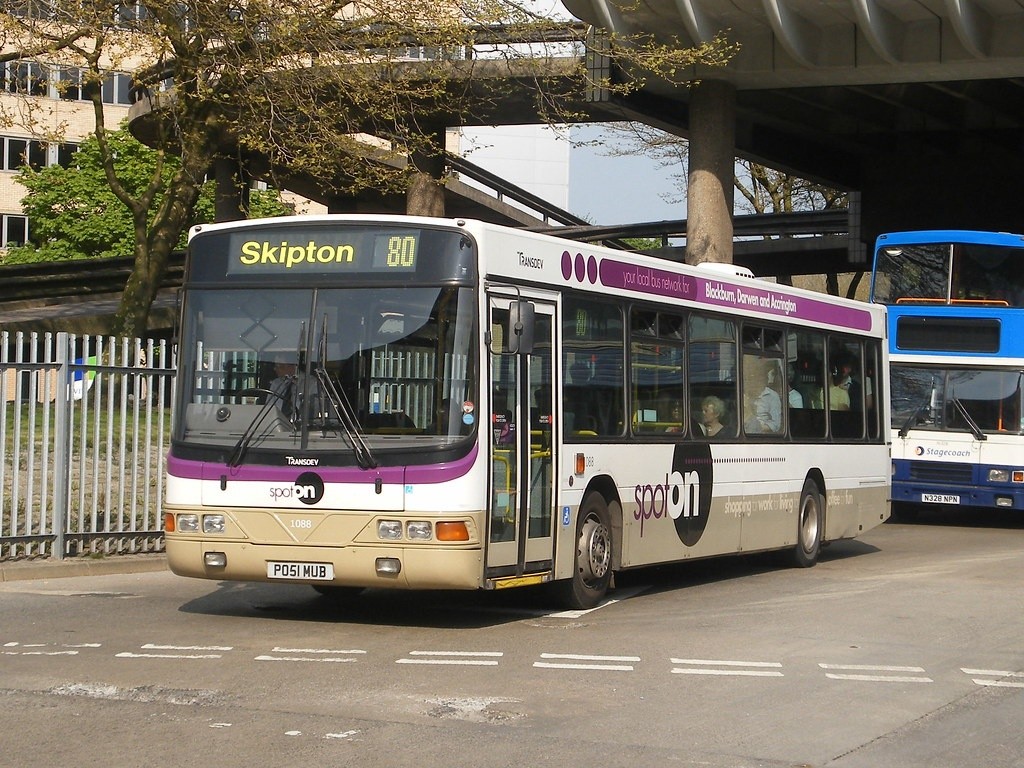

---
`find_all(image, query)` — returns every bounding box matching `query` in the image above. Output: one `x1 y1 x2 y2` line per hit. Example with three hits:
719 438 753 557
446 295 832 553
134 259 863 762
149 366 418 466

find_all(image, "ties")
281 382 293 419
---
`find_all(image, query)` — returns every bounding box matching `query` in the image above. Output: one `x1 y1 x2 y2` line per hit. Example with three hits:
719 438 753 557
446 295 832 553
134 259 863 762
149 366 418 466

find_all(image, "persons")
425 398 468 435
266 352 320 419
701 396 735 437
564 359 616 435
789 359 872 410
664 398 704 435
744 368 781 433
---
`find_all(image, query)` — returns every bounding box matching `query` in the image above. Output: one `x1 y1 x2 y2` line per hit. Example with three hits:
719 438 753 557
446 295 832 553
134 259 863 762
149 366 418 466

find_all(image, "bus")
867 228 1024 513
159 212 894 613
